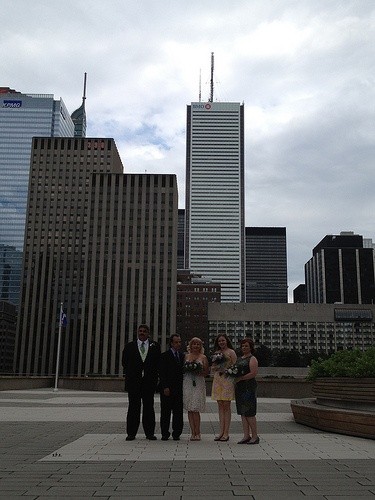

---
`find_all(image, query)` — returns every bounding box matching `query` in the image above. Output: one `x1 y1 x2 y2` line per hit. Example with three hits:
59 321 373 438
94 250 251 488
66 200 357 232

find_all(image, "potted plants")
306 347 375 406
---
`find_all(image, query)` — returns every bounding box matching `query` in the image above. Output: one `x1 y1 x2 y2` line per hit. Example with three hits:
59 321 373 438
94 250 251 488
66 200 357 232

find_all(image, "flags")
62 309 68 326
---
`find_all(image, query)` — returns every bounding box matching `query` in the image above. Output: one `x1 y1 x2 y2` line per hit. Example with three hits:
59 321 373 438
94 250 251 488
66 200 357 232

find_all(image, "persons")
183 337 209 440
235 338 260 445
160 334 186 441
122 325 160 441
211 334 237 442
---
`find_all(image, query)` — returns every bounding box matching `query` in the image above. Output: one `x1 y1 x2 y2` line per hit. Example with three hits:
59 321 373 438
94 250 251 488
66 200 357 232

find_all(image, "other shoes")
173 436 180 440
219 435 229 441
246 437 259 444
190 435 196 441
126 434 135 440
195 435 201 441
161 436 169 440
237 436 251 444
214 434 223 441
146 434 157 440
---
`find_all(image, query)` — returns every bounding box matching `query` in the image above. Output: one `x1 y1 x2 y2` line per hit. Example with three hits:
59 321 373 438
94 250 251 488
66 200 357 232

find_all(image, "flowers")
184 359 202 373
211 351 229 369
150 342 154 347
221 362 247 382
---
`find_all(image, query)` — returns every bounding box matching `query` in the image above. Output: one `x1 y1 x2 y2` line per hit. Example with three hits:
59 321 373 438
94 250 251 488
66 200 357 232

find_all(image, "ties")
174 352 179 361
140 343 145 354
